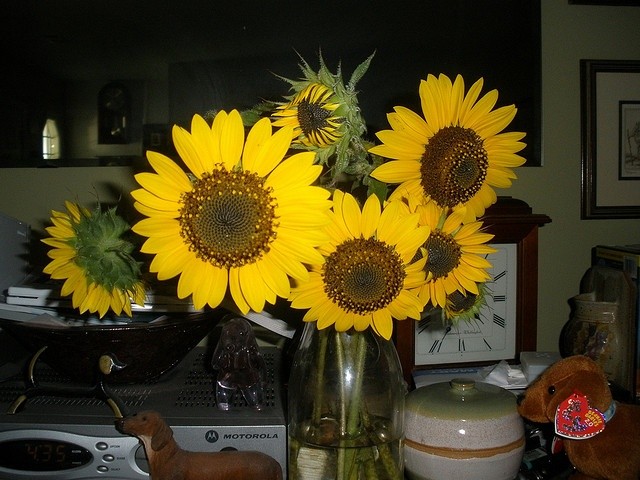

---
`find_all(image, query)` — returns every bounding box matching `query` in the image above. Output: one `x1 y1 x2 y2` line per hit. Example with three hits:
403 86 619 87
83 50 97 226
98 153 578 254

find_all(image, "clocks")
401 191 550 377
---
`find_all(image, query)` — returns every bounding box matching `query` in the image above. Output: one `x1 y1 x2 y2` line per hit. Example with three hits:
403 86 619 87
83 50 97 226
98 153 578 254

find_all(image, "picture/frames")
579 60 638 221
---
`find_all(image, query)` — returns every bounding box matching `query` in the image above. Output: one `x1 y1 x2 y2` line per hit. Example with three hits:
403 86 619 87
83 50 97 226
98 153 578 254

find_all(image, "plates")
0 308 225 383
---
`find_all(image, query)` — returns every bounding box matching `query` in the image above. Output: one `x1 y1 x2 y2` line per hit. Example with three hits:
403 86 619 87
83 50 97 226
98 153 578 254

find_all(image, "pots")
404 378 525 480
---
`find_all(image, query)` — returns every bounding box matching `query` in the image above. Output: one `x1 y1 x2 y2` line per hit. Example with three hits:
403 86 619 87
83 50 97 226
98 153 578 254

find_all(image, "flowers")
45 42 528 466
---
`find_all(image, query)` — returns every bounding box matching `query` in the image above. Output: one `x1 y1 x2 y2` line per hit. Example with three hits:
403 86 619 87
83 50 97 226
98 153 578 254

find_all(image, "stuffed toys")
517 354 639 479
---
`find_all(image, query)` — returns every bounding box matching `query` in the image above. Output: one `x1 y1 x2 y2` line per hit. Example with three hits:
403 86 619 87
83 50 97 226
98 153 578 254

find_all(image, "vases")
285 308 406 480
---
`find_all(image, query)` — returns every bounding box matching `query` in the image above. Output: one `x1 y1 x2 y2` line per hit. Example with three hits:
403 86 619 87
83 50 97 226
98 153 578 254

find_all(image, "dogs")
212 319 266 410
114 410 283 480
